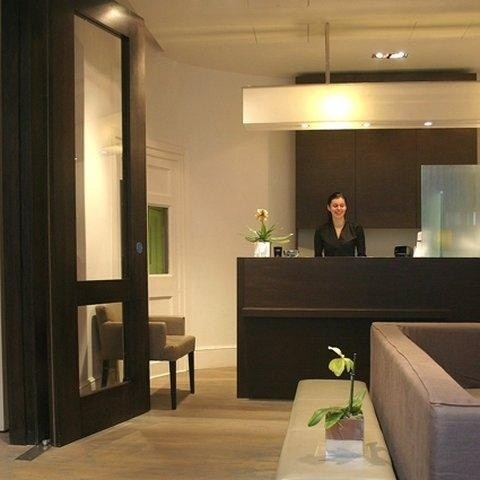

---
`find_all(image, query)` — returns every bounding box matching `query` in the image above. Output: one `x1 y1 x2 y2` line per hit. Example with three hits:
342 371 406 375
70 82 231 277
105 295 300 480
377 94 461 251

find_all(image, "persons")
314 192 366 257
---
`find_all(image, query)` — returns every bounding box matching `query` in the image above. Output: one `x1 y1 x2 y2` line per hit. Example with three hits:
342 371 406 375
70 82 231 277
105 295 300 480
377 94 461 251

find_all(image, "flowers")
237 208 294 244
308 345 367 429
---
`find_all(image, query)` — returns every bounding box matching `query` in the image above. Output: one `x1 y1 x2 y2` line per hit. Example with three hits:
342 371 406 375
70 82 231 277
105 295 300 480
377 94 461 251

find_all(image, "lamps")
102 143 124 156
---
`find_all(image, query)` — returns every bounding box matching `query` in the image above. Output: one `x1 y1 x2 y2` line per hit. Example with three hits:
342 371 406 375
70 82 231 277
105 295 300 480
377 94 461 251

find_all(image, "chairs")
95 302 196 408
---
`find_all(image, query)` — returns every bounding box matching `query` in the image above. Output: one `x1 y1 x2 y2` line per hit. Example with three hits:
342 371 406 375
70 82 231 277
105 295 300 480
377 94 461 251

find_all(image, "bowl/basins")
283 249 301 258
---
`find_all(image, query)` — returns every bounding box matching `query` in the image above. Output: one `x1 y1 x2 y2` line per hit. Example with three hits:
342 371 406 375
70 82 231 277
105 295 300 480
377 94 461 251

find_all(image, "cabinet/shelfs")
294 70 478 227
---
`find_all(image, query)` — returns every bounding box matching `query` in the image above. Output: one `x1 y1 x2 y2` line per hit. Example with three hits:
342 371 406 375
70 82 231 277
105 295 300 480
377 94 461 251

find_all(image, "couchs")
369 321 480 480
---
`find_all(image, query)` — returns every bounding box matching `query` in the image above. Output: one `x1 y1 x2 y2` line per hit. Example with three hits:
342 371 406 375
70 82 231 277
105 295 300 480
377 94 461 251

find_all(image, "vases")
253 242 271 258
324 415 364 464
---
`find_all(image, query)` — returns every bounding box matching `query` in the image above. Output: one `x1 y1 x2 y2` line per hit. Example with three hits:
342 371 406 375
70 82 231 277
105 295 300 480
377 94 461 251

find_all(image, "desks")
276 380 397 480
